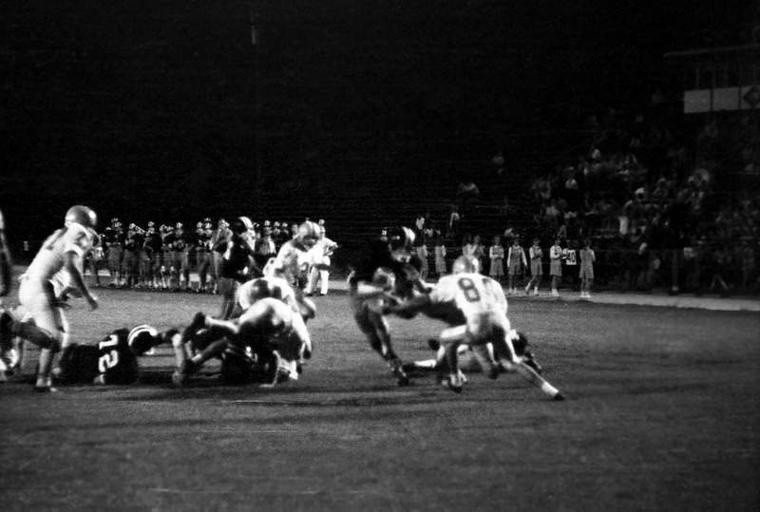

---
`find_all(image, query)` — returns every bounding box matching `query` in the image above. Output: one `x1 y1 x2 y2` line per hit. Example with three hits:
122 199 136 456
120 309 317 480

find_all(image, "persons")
345 225 565 402
0 203 338 394
409 148 760 299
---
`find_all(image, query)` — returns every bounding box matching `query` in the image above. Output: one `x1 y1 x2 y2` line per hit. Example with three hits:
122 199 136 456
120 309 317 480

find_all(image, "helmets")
238 216 252 229
372 266 396 289
127 324 156 355
452 254 479 273
221 354 251 382
251 279 268 297
296 221 320 249
65 205 98 227
387 226 415 263
505 329 526 354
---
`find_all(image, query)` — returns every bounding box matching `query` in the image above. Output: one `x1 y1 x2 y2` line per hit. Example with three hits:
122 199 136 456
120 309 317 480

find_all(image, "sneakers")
183 312 205 343
179 360 199 374
552 392 563 400
1 309 16 349
33 386 57 392
157 329 177 344
387 339 462 392
